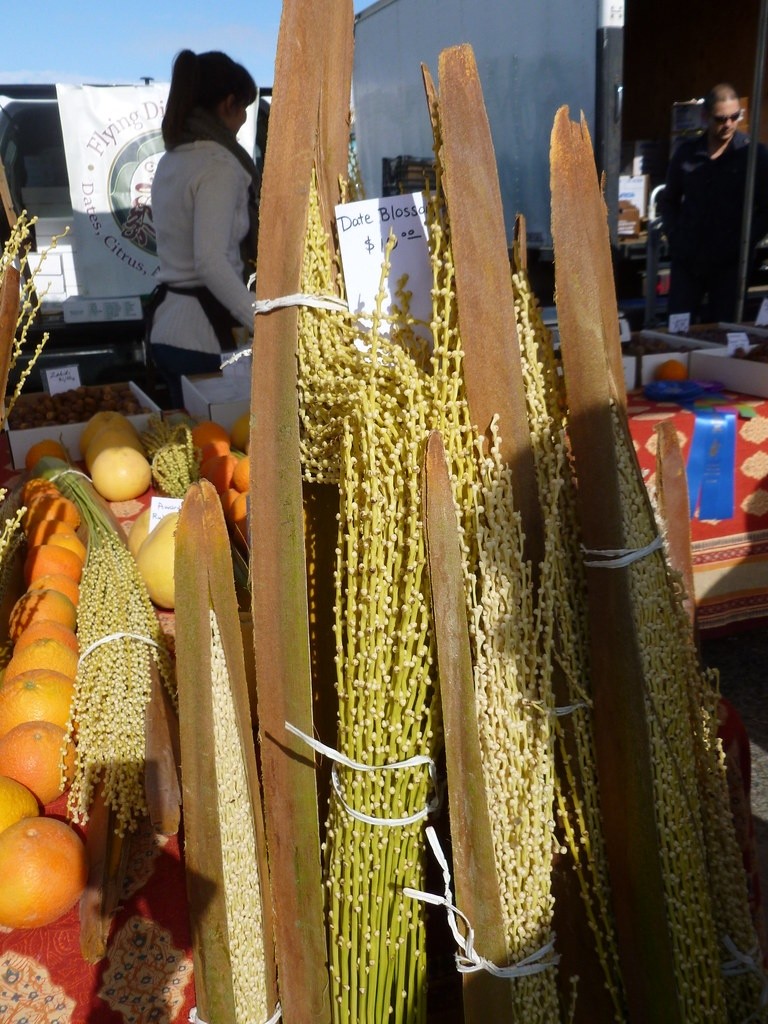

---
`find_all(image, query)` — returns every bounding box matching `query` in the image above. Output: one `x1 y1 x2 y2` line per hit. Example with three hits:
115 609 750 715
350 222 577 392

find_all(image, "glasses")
709 111 740 125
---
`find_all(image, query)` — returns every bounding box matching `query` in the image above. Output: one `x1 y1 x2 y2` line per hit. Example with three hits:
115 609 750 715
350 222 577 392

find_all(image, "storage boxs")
641 321 768 348
41 365 83 396
555 354 635 392
688 344 768 398
221 348 254 375
2 381 164 470
180 361 254 435
623 331 727 388
61 294 144 323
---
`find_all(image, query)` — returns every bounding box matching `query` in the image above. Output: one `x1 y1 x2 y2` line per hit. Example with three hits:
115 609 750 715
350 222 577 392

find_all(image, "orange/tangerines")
0 437 89 927
653 359 686 380
187 420 249 545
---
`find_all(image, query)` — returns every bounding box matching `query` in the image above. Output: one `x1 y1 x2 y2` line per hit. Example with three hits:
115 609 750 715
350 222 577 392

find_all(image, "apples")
77 412 179 609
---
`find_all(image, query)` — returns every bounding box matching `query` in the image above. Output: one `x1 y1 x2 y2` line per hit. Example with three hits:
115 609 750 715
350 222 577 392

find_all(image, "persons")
140 47 258 419
656 81 768 331
